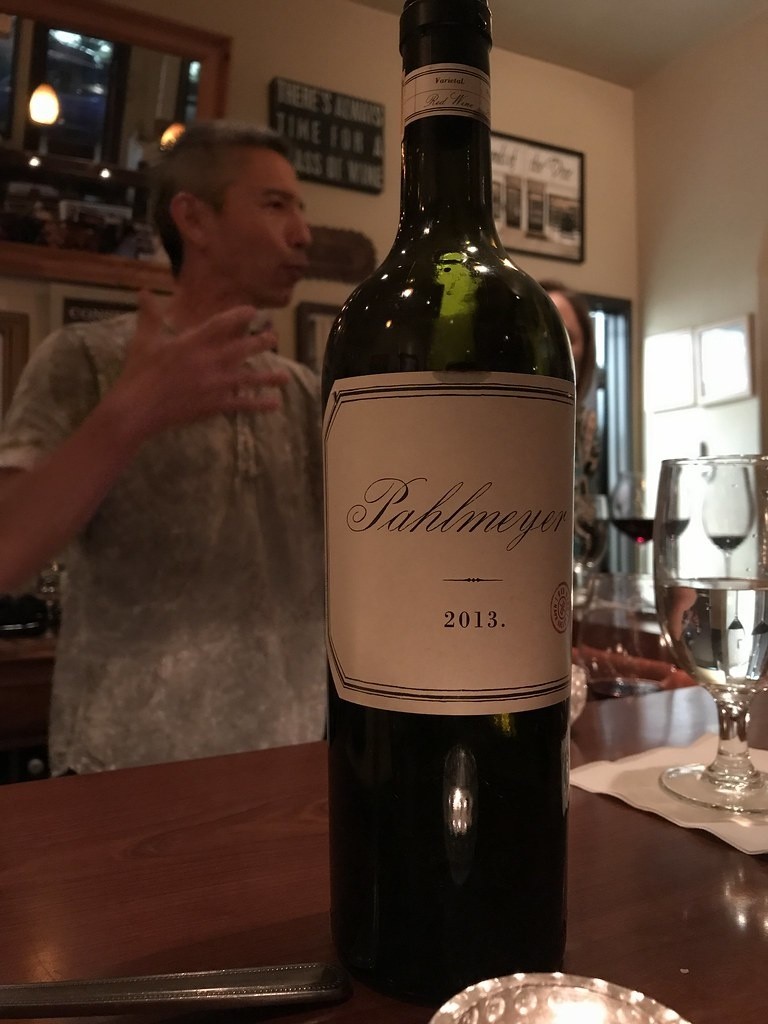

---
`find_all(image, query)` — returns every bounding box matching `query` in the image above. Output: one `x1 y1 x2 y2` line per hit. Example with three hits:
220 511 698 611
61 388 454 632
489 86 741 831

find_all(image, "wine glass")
701 468 753 580
661 483 692 584
654 453 768 813
572 492 607 588
723 590 745 664
750 590 768 657
607 471 654 574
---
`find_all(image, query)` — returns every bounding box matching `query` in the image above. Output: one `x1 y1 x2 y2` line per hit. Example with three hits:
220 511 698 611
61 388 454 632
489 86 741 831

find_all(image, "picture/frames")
647 313 753 415
492 129 585 263
1 0 234 296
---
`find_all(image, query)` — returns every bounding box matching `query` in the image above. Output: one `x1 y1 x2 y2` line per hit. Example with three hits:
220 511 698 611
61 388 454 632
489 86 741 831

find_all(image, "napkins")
568 729 767 857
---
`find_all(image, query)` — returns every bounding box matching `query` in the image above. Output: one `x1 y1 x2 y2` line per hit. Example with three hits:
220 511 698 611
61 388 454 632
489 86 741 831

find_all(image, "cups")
428 972 688 1024
578 570 669 696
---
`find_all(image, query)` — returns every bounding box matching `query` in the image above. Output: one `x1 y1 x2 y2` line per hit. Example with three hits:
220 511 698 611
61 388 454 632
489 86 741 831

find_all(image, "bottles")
329 0 577 1024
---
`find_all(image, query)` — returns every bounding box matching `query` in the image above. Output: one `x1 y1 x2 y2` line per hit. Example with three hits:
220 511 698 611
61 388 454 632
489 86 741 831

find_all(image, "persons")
530 275 603 562
0 117 334 777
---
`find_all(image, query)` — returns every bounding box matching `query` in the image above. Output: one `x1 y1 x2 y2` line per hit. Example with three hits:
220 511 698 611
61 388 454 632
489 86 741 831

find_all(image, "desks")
1 683 768 1024
